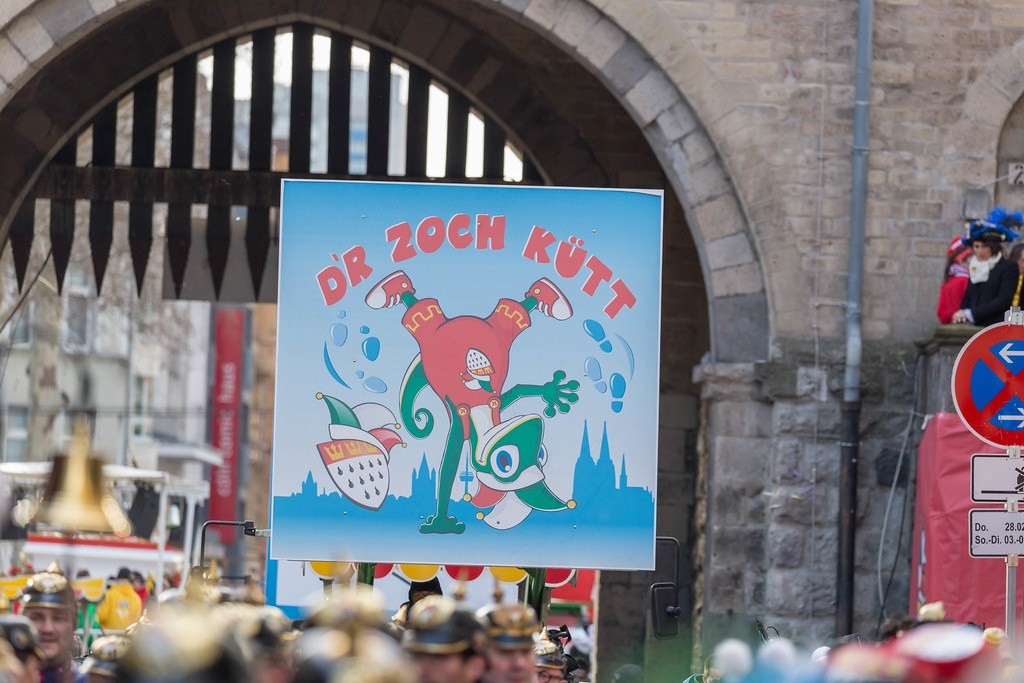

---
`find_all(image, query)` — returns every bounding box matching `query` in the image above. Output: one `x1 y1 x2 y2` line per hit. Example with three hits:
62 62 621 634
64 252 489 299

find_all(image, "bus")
0 460 197 606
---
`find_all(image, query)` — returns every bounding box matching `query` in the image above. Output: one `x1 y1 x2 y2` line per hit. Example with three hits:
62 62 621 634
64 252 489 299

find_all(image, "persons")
0 566 594 683
935 207 1024 324
686 598 1024 683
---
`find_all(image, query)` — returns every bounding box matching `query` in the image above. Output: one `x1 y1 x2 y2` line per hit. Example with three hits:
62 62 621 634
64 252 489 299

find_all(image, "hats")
124 558 281 683
17 561 76 608
401 594 483 653
487 603 541 650
530 626 565 668
0 615 38 662
291 584 422 683
408 576 444 601
960 207 1022 242
947 237 973 263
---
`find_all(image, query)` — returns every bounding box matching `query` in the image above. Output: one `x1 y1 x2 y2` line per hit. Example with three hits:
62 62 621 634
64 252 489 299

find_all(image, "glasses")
534 670 569 683
